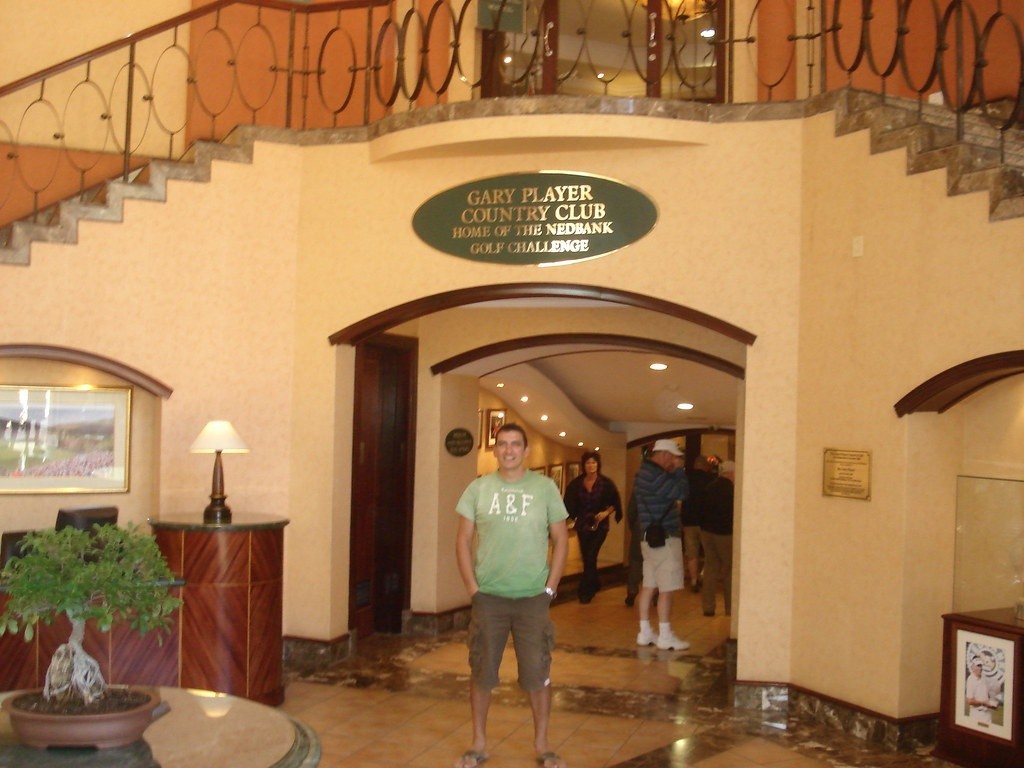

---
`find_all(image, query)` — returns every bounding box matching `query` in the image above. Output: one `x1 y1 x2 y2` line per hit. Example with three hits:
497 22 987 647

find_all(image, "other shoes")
625 593 637 606
651 592 660 606
692 580 700 592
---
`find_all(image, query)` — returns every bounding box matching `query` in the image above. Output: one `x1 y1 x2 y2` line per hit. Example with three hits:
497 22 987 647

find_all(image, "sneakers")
656 635 691 651
637 629 659 645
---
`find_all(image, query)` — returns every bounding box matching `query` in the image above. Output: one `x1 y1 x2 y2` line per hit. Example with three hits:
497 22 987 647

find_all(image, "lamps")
189 419 250 523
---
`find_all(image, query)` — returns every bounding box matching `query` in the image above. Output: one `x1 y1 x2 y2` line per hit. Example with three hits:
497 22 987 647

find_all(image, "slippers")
457 749 489 768
537 751 562 768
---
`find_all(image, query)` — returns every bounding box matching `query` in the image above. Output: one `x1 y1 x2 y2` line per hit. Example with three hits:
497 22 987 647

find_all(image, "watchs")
545 585 557 600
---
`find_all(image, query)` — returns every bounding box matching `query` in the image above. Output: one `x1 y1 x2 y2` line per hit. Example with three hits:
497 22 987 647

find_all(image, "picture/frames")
529 466 545 477
566 462 583 488
486 409 507 448
0 385 134 495
547 463 563 495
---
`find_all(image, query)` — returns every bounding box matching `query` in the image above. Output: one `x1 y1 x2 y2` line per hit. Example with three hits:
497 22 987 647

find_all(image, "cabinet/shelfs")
929 605 1024 768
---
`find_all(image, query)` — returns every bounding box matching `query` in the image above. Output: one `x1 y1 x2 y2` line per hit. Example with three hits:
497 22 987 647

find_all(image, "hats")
652 439 686 455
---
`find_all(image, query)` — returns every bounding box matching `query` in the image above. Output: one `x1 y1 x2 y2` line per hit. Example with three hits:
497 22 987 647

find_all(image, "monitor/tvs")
54 506 118 565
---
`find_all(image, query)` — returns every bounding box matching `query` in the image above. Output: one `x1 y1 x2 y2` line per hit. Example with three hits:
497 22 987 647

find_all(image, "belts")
642 532 675 540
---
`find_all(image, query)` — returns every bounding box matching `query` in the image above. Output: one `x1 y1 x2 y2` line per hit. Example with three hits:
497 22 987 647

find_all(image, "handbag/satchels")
646 523 665 548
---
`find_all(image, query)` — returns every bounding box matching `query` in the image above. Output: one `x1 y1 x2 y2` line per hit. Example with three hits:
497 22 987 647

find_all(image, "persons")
636 439 690 651
624 447 732 615
456 421 571 768
965 655 998 725
562 451 623 602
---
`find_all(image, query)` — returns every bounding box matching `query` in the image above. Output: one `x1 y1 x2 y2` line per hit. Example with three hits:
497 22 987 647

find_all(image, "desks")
0 684 322 768
149 512 290 707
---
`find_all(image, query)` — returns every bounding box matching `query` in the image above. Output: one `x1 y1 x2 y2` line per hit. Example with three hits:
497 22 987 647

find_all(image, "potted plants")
0 520 185 751
